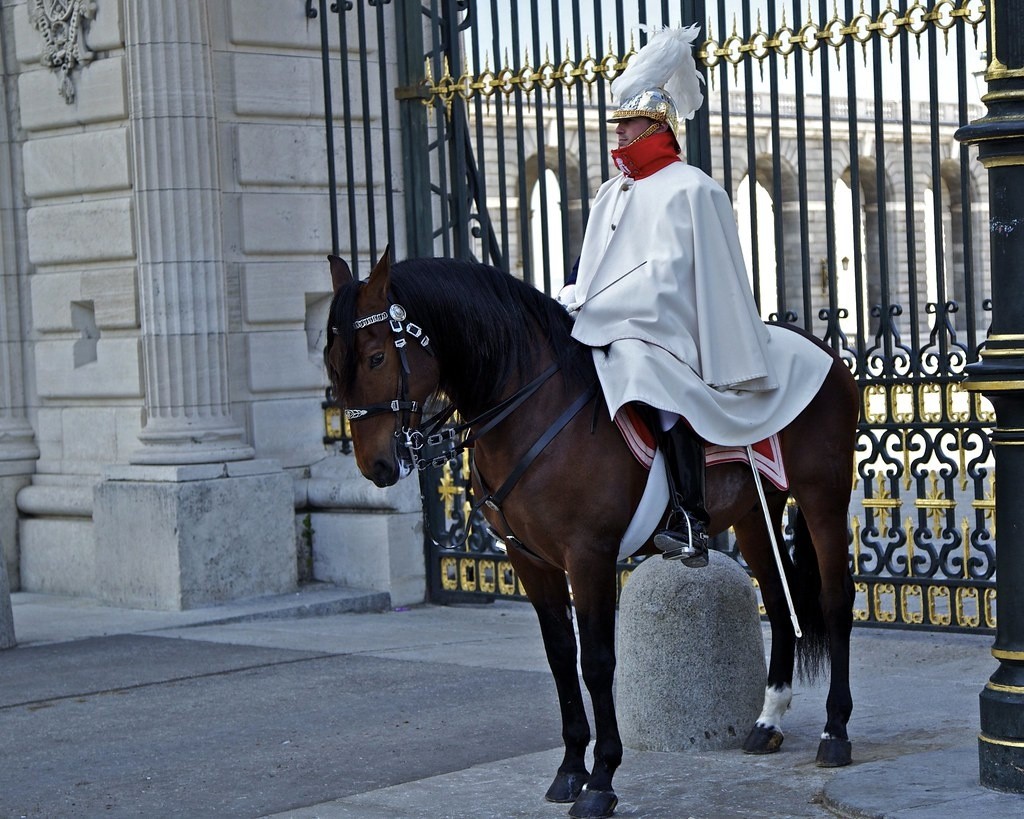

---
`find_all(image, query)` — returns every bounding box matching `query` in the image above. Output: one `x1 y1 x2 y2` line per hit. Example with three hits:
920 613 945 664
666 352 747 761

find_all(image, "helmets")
607 86 681 155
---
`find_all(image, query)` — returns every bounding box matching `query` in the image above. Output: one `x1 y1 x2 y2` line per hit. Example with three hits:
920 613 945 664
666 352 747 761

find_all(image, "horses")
322 244 861 819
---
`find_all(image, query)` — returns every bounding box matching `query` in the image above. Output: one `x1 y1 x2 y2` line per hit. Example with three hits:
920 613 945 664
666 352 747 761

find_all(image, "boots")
654 420 711 568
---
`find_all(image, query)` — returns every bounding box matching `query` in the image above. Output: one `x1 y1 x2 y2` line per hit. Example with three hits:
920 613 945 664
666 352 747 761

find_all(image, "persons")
560 23 765 569
